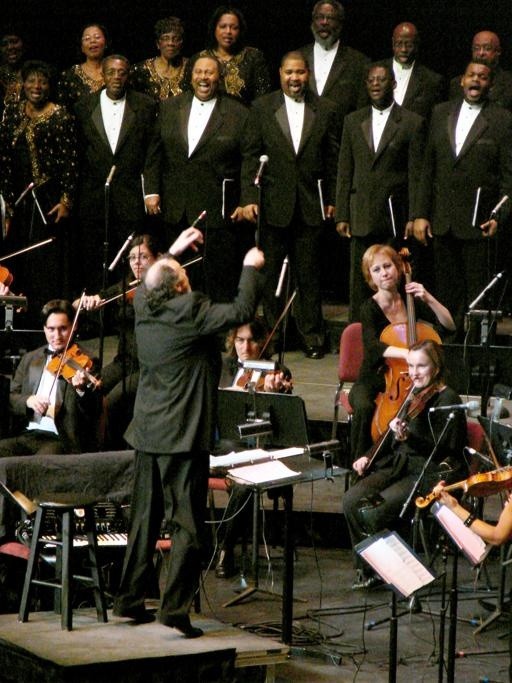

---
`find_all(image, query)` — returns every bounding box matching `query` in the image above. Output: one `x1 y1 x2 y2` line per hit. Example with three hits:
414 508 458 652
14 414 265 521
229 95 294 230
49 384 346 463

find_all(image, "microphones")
106 224 143 276
15 177 41 207
463 265 512 311
250 149 274 189
425 398 484 415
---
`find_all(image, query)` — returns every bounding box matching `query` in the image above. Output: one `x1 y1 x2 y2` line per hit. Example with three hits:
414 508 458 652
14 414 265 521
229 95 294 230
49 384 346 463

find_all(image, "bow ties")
234 360 243 368
43 347 57 355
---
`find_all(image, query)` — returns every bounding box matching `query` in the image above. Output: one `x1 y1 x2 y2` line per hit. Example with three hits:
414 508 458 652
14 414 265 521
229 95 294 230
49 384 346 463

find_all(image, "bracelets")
463 512 475 527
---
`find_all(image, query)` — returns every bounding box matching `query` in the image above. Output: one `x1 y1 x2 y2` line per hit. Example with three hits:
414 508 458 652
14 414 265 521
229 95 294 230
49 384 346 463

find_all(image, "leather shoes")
113 607 156 622
159 616 203 638
215 550 230 578
352 569 386 592
305 345 321 359
74 327 90 342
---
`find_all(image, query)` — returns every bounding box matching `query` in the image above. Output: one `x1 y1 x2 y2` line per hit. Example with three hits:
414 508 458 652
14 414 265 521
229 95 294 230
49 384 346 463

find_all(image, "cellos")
371 248 445 445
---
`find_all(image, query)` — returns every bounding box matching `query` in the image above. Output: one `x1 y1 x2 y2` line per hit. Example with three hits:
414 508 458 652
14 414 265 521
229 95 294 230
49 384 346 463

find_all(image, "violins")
415 466 512 509
235 368 293 394
46 344 104 391
395 384 436 440
1 268 27 313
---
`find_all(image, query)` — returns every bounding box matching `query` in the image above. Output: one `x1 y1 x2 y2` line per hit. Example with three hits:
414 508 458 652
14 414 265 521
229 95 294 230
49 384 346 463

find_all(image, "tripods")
220 438 311 611
363 502 481 630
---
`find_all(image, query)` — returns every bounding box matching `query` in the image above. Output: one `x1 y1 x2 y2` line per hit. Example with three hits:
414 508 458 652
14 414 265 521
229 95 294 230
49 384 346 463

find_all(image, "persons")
79 55 162 284
413 57 511 341
71 233 159 452
186 2 270 111
346 244 466 485
447 28 509 108
430 474 512 552
360 20 441 135
144 55 260 304
57 22 117 124
110 226 266 639
338 338 468 591
1 297 104 458
1 29 27 94
204 317 295 577
133 16 193 99
334 61 424 323
0 57 77 326
239 51 340 358
294 0 373 152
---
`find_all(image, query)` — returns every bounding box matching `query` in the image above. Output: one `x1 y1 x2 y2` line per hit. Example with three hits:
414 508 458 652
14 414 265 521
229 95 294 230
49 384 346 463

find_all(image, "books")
359 533 436 597
436 504 486 564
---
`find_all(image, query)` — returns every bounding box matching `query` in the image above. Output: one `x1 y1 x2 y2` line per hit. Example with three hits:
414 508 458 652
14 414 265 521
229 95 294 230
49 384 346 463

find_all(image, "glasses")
126 254 158 262
82 34 105 42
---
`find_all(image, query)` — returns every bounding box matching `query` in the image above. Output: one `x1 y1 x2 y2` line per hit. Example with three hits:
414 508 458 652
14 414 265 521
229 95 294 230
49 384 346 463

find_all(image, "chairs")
330 320 384 466
408 420 492 590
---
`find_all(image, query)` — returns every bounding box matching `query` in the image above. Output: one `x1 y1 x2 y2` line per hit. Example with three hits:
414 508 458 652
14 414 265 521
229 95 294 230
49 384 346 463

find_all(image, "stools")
206 477 248 581
0 540 45 566
151 538 203 616
18 496 111 631
